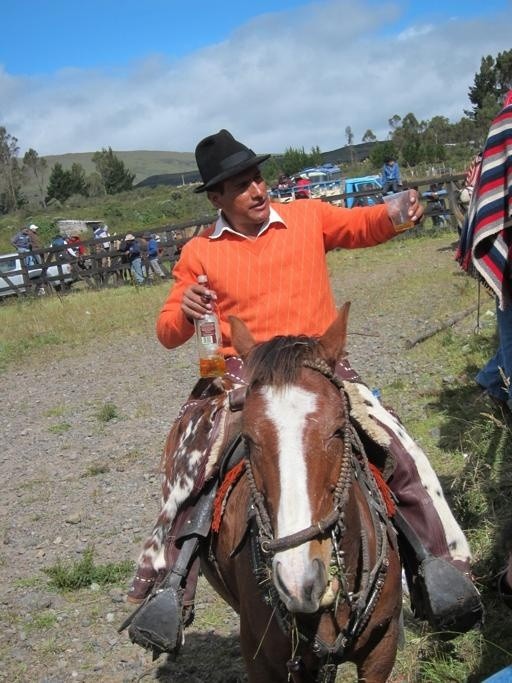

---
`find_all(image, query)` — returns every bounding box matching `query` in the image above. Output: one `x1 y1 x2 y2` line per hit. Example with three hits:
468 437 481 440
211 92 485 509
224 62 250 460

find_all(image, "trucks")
262 176 384 206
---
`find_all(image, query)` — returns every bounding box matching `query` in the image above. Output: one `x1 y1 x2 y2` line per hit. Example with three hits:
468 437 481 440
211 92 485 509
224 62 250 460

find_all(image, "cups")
382 190 414 232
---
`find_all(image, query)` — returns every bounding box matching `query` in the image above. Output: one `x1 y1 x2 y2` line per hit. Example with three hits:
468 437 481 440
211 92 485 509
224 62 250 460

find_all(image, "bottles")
193 275 227 379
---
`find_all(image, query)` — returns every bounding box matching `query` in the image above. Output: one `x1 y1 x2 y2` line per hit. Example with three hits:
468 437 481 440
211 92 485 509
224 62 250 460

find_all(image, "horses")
450 186 474 237
164 300 403 683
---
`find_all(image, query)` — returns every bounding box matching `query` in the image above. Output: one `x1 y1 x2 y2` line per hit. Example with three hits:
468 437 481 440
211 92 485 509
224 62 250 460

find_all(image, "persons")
461 90 512 406
269 163 317 198
380 153 405 195
12 220 184 287
156 129 426 355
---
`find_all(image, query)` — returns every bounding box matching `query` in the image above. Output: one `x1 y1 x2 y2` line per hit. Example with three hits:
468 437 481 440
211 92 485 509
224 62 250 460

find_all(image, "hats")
125 234 135 241
29 225 39 229
193 129 271 193
70 233 80 237
142 232 153 238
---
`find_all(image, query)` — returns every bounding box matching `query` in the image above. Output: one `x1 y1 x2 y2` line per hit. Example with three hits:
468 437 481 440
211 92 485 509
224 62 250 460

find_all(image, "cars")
0 250 78 307
